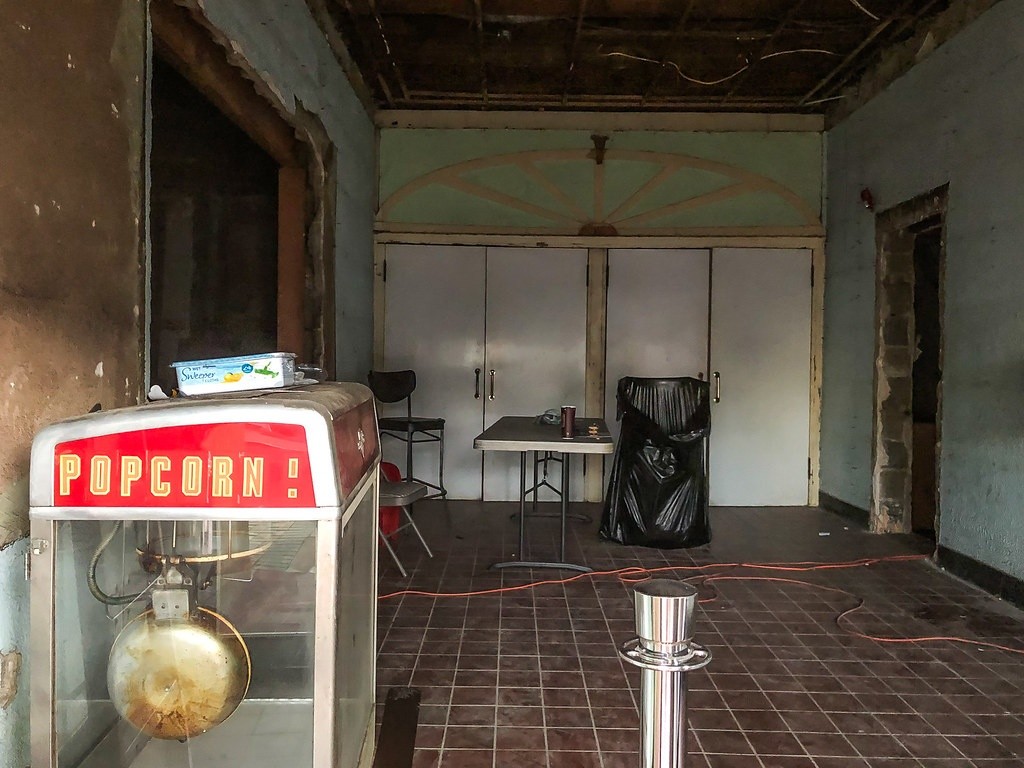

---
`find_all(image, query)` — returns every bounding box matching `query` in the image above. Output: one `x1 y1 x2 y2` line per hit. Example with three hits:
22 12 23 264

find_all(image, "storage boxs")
168 352 296 397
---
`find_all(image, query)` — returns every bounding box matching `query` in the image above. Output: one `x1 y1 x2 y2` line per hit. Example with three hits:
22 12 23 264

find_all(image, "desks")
473 417 614 574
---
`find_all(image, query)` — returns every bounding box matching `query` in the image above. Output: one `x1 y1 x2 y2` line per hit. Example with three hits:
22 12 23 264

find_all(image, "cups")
561 405 576 439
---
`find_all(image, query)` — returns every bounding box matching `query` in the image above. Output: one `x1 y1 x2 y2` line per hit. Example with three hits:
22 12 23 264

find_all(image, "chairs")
367 369 447 515
378 468 433 578
